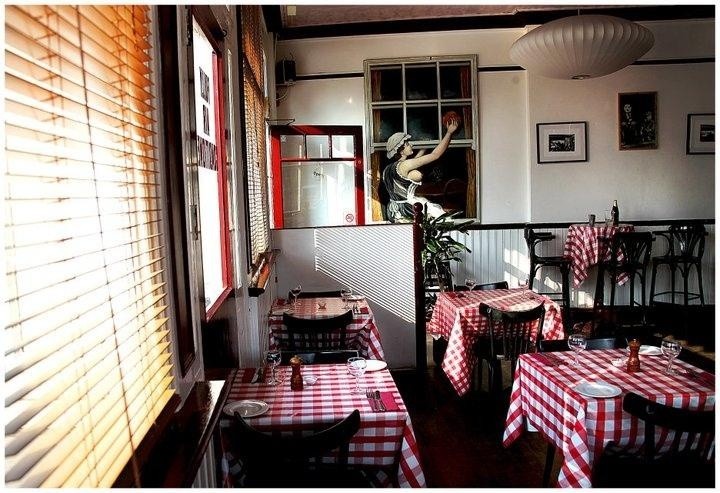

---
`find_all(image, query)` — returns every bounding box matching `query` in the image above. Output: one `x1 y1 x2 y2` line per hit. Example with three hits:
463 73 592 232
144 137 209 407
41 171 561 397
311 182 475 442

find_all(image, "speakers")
276 59 297 84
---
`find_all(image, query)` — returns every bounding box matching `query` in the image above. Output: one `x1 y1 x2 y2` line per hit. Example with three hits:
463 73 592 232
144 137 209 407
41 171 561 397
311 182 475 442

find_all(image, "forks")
365 387 379 412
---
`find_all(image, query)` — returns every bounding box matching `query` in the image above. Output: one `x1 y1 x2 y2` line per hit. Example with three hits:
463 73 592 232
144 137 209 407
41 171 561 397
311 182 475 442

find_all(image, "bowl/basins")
303 373 319 386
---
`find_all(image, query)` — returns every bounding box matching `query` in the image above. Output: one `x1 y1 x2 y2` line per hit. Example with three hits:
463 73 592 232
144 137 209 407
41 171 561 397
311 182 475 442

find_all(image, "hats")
386 132 412 159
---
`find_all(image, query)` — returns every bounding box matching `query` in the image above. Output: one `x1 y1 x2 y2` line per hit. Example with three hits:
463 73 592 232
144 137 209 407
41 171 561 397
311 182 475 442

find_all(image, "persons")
382 117 459 224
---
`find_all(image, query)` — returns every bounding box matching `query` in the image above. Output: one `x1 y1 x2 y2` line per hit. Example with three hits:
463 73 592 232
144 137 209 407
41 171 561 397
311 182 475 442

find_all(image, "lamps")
508 10 654 80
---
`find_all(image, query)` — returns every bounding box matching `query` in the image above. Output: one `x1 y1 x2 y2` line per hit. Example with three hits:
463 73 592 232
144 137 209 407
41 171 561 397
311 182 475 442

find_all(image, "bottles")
626 338 640 372
290 354 303 391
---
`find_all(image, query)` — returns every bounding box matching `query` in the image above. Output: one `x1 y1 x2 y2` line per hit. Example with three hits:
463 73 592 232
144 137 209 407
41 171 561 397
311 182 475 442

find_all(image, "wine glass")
567 333 588 366
261 349 283 386
289 282 302 307
518 277 530 299
340 287 352 309
347 356 367 395
660 338 683 374
465 277 478 297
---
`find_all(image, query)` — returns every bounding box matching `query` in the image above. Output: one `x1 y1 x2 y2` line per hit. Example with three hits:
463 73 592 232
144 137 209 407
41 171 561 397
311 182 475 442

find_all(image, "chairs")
594 221 710 319
521 221 574 314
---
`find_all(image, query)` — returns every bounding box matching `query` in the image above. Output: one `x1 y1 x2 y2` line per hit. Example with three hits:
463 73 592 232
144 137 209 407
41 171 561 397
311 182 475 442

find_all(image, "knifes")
375 390 386 411
252 367 260 383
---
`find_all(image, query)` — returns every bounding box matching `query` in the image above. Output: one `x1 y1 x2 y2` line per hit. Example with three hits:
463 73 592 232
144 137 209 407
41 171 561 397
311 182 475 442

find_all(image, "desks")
568 222 634 316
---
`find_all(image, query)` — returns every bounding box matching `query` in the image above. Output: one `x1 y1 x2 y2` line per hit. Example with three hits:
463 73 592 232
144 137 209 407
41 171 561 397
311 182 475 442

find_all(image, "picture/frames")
536 121 587 164
685 111 714 154
617 89 660 150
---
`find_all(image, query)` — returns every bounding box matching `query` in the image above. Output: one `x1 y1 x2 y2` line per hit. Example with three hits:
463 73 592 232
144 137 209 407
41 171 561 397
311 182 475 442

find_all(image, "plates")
222 398 269 418
573 381 622 397
346 294 366 300
350 358 387 371
627 344 662 355
